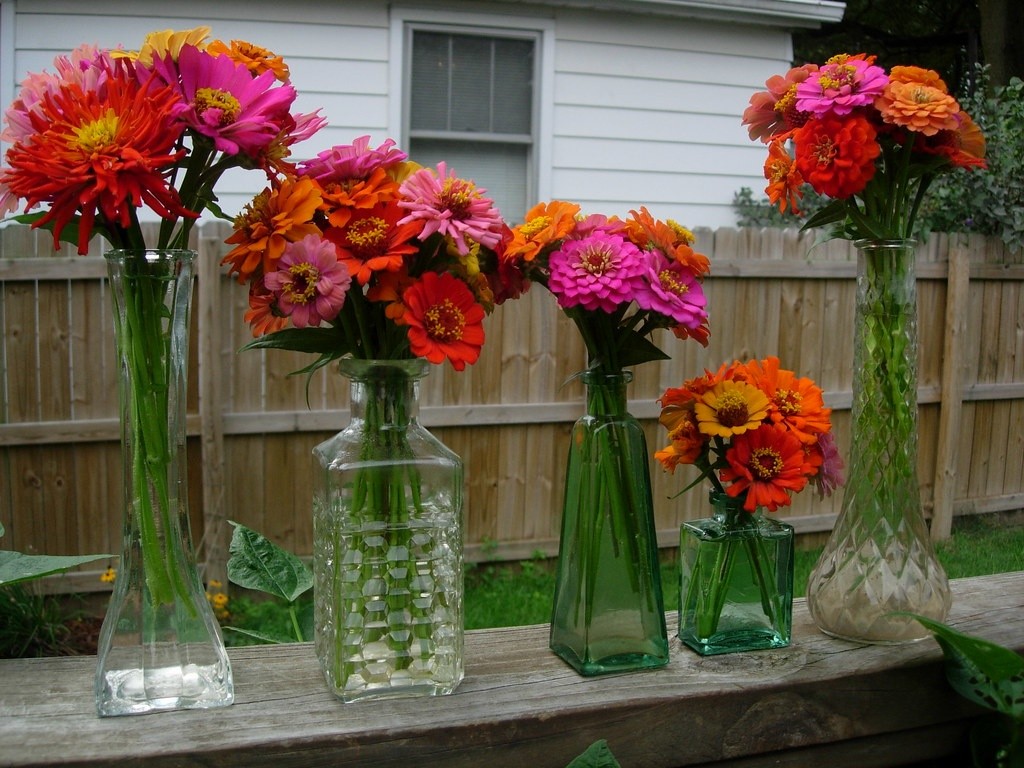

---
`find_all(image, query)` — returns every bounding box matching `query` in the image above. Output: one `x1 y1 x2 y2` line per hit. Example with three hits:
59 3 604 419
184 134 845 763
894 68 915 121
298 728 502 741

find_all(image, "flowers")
741 53 989 587
219 134 530 689
0 26 330 616
502 199 711 628
655 353 845 635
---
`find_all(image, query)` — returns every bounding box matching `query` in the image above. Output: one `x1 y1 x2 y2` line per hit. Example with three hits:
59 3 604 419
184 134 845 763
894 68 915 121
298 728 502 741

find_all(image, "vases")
96 249 236 718
549 371 670 676
677 488 794 656
312 357 465 705
806 237 953 645
